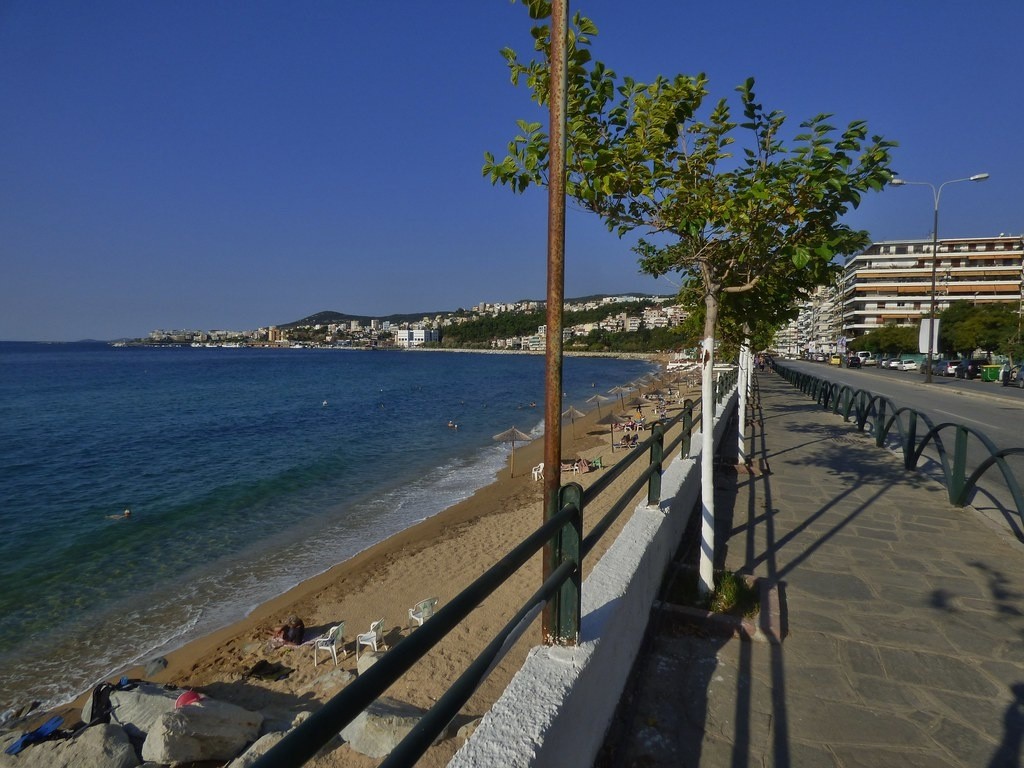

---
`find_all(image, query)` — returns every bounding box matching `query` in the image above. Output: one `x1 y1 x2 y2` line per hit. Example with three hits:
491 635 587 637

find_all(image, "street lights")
887 172 991 384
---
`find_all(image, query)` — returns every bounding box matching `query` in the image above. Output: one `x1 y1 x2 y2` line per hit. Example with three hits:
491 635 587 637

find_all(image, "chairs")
357 617 388 662
532 463 545 481
561 455 602 474
315 621 346 667
408 595 440 634
612 378 702 450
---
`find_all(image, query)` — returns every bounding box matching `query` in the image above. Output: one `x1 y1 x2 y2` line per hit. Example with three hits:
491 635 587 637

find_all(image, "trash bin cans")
981 365 1000 382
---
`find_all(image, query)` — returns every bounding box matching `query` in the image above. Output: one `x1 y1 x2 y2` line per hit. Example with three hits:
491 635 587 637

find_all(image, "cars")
1003 363 1024 388
863 357 877 366
847 356 862 368
897 358 917 371
932 359 961 377
828 355 842 365
919 357 942 374
881 357 888 367
796 354 829 361
885 356 902 369
954 358 990 380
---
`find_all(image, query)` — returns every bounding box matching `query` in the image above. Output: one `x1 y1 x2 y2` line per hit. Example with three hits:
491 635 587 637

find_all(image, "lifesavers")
175 691 200 707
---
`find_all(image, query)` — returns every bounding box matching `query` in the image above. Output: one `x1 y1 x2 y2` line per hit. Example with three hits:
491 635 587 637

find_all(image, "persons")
627 416 633 425
621 433 638 445
266 615 305 645
636 403 642 413
755 353 774 374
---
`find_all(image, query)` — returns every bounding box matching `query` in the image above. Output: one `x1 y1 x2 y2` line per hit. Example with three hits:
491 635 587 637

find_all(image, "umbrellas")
586 394 609 419
608 368 700 410
562 406 586 441
492 425 532 479
596 410 627 452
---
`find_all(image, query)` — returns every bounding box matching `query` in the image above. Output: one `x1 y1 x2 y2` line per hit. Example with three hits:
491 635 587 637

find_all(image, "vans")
855 351 872 364
